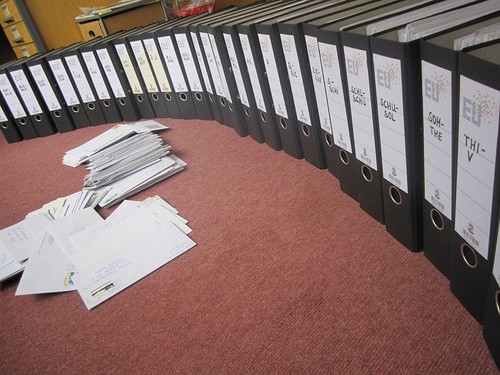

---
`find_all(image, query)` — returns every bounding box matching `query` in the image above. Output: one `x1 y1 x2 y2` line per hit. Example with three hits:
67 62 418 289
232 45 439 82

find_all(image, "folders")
0 1 500 373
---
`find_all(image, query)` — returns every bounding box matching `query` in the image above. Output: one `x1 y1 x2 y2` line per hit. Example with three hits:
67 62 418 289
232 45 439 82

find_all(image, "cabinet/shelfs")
0 0 120 60
74 0 167 41
